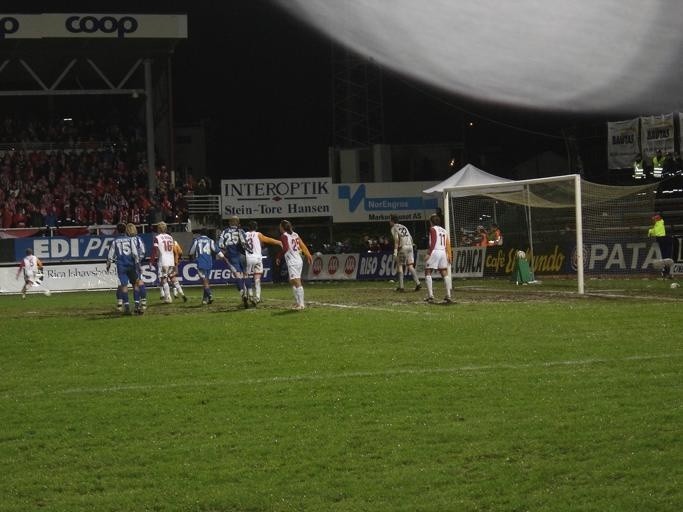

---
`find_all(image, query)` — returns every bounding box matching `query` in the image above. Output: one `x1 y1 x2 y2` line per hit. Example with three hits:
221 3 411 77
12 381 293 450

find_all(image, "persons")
219 216 256 306
188 229 228 306
0 112 212 234
631 149 683 184
113 223 147 312
16 248 50 298
106 223 142 315
149 220 187 303
389 216 422 292
244 220 283 304
160 232 183 299
424 214 453 304
276 220 313 310
647 215 666 237
302 223 502 256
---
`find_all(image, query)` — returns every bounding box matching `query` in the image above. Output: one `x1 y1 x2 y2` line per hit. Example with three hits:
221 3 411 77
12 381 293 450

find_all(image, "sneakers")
114 296 213 316
395 284 456 303
21 285 30 298
242 296 306 312
